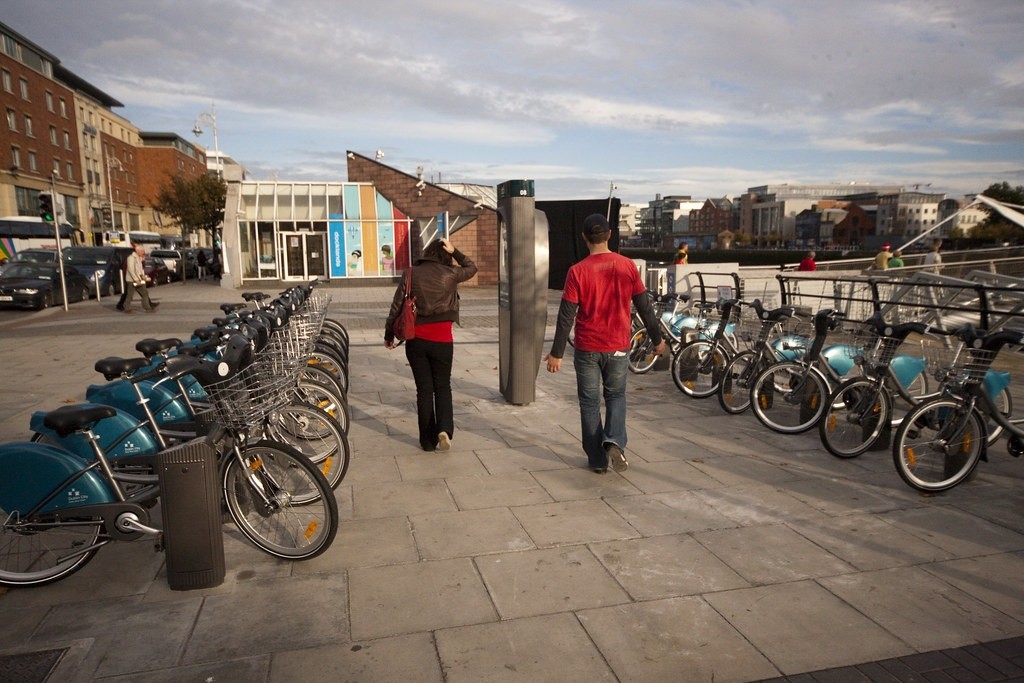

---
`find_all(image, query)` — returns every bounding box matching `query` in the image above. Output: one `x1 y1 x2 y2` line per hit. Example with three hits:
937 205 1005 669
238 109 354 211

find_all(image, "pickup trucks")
150 250 197 283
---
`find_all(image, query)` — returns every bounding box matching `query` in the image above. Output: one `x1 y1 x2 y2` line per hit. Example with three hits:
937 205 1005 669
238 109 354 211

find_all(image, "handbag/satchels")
394 264 417 341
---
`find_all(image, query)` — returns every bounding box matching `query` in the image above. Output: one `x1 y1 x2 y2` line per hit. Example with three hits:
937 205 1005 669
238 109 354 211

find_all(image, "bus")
102 231 162 254
0 215 85 265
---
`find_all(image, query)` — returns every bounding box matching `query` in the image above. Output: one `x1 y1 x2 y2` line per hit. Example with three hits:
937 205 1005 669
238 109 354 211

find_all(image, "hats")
879 242 890 249
582 212 610 235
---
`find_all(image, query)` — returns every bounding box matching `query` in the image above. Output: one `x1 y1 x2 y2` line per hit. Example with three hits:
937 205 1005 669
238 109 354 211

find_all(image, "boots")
116 293 126 310
148 297 159 308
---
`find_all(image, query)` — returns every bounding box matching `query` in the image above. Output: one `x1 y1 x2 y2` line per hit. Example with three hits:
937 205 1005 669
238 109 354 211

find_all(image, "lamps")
417 181 426 190
474 202 484 210
348 152 355 159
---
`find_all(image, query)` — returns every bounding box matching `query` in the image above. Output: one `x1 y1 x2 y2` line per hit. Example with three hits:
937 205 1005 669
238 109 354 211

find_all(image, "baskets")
653 301 677 319
843 327 901 368
739 317 776 344
776 320 816 350
696 308 722 332
922 339 997 386
205 293 332 428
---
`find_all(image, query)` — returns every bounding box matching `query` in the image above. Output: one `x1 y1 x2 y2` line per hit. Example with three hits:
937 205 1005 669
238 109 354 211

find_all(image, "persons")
888 251 903 268
673 242 688 264
542 213 665 474
798 251 816 271
383 237 478 452
875 242 893 269
197 250 208 282
214 243 222 279
116 244 160 313
924 239 945 264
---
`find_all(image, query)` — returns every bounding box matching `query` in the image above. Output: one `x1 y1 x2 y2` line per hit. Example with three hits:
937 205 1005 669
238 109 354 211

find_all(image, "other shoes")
590 462 608 474
122 309 136 314
145 308 158 312
606 442 629 472
438 431 451 451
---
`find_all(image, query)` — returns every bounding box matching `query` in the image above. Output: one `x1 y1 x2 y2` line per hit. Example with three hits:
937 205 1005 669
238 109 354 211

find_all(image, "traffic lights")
47 176 53 187
38 193 53 221
103 208 112 228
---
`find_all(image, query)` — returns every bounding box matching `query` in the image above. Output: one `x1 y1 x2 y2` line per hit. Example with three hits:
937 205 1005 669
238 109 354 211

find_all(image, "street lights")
191 112 220 180
107 158 124 231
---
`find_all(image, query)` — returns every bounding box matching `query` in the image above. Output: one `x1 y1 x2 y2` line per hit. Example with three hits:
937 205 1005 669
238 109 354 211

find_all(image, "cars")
190 247 222 275
143 258 171 288
0 247 132 311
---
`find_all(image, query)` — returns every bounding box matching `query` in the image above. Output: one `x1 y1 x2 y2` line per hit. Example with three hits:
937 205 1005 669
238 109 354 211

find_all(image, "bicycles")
892 322 1024 494
818 313 1011 460
718 299 812 413
748 310 928 435
569 290 814 399
0 279 350 589
